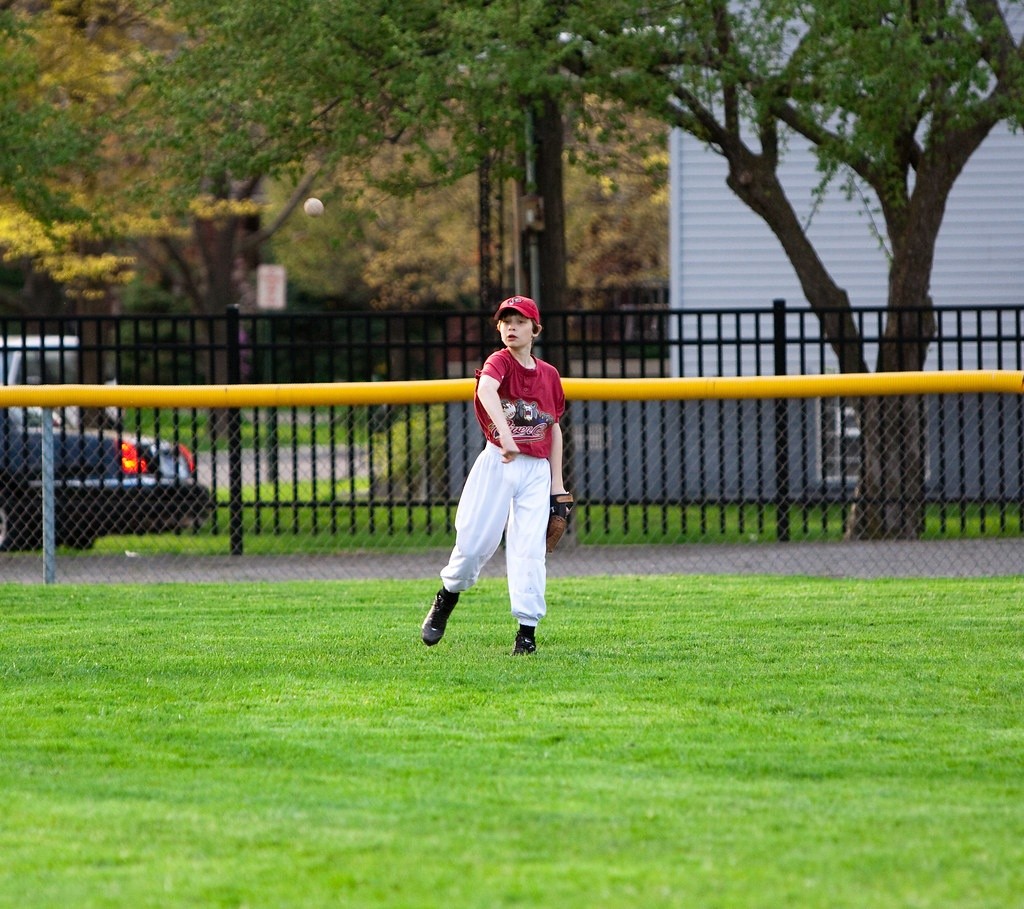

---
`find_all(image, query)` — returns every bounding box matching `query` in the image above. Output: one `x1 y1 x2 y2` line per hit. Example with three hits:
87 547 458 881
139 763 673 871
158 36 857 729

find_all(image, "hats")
494 295 540 325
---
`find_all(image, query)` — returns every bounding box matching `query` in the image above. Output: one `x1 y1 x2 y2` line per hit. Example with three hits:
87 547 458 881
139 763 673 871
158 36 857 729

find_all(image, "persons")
421 295 572 656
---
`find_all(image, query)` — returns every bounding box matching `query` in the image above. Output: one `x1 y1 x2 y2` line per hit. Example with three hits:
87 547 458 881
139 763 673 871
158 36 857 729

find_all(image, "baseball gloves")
546 490 576 554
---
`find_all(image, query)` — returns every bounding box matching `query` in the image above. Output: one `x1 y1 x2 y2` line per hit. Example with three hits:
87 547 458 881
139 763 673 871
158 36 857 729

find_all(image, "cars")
0 407 210 550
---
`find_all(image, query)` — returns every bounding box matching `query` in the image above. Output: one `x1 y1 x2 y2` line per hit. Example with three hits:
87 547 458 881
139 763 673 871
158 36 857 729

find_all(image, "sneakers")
509 630 536 656
421 590 458 646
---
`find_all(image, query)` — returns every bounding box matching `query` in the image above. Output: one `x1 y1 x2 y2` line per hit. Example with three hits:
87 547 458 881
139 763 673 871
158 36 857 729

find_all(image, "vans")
0 335 125 429
